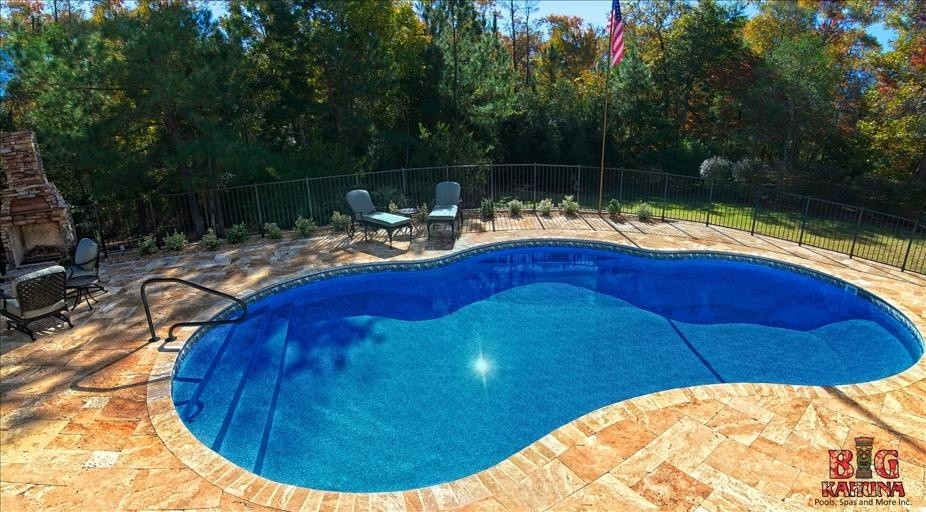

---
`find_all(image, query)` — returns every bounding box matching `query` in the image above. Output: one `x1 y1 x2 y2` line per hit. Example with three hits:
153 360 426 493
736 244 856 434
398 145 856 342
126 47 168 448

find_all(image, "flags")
607 0 624 68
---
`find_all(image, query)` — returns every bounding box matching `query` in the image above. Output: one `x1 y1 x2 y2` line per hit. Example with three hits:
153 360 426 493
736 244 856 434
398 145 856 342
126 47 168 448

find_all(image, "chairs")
342 189 413 249
0 236 100 342
425 181 464 244
